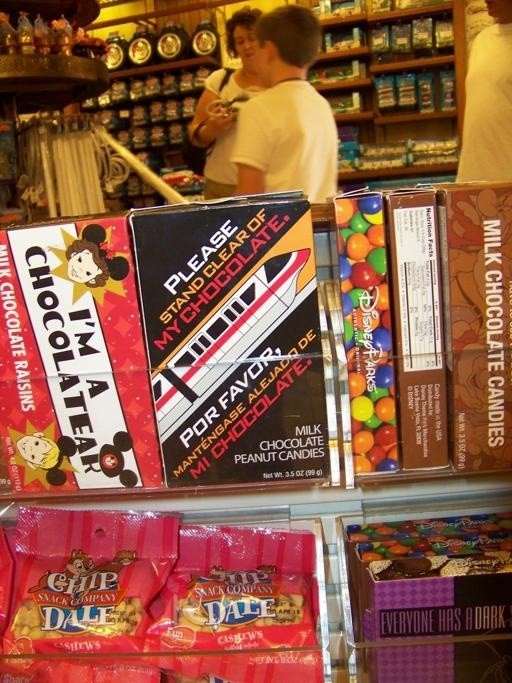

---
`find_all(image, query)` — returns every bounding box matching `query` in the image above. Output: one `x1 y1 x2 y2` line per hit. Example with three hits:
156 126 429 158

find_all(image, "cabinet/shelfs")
2 1 107 225
83 0 375 211
357 0 467 190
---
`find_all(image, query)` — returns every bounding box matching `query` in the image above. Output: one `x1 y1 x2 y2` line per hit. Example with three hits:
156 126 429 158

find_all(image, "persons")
188 6 265 199
230 5 338 203
455 0 512 184
